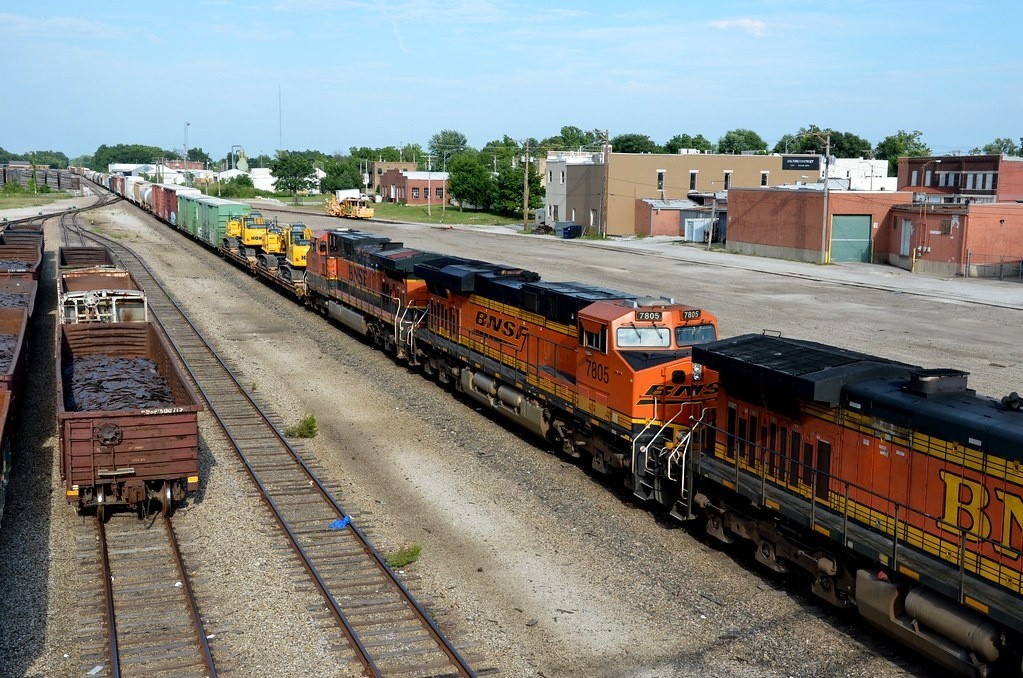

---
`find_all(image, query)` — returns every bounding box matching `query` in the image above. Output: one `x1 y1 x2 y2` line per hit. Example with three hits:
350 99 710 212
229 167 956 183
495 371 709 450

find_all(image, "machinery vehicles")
237 213 267 257
222 213 243 249
278 222 314 282
257 221 288 270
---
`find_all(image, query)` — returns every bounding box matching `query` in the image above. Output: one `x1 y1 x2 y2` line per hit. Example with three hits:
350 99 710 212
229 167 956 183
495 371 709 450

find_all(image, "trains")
68 166 1022 677
55 245 204 523
0 222 45 451
